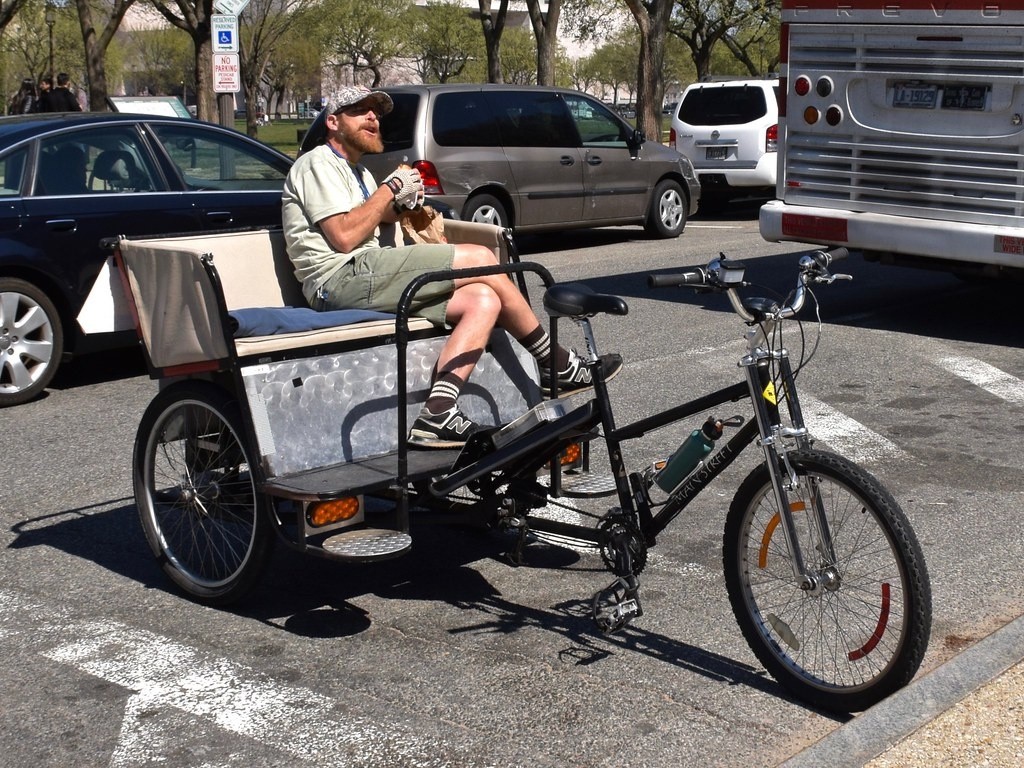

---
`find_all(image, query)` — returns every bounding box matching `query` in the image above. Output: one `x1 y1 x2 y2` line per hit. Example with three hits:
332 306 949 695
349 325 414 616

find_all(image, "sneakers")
540 349 623 400
407 404 497 447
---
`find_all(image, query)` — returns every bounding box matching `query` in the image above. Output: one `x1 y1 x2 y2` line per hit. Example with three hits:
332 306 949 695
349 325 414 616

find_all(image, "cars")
0 113 466 404
609 102 637 119
663 102 678 115
300 108 320 117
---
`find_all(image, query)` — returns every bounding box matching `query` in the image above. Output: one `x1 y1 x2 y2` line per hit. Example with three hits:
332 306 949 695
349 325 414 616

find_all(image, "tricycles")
101 217 937 719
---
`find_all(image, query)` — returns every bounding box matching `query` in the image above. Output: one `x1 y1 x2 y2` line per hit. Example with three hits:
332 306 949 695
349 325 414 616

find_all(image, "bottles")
653 416 745 494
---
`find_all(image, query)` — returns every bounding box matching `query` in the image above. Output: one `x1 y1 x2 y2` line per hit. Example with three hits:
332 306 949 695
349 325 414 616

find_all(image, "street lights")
43 1 62 89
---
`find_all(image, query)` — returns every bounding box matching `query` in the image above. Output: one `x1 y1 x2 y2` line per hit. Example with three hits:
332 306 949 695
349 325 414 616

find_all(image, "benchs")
77 219 524 377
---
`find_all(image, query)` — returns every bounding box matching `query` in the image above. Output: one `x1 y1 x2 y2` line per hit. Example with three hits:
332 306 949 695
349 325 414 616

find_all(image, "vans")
296 82 702 244
671 71 778 201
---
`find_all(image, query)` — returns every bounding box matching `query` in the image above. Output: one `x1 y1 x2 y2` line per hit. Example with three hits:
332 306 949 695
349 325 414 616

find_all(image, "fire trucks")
760 2 1024 286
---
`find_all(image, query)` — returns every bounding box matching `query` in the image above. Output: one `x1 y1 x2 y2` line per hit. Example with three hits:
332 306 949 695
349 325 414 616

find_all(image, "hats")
326 86 393 116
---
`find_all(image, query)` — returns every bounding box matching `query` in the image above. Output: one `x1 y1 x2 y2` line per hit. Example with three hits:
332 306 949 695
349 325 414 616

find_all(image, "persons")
256 113 269 127
4 73 152 191
281 84 623 448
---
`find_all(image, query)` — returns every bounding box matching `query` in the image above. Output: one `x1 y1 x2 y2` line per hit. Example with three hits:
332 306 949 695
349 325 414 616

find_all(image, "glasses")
336 105 384 118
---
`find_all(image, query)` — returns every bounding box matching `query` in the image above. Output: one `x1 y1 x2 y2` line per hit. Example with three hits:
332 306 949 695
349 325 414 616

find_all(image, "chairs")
94 152 134 191
